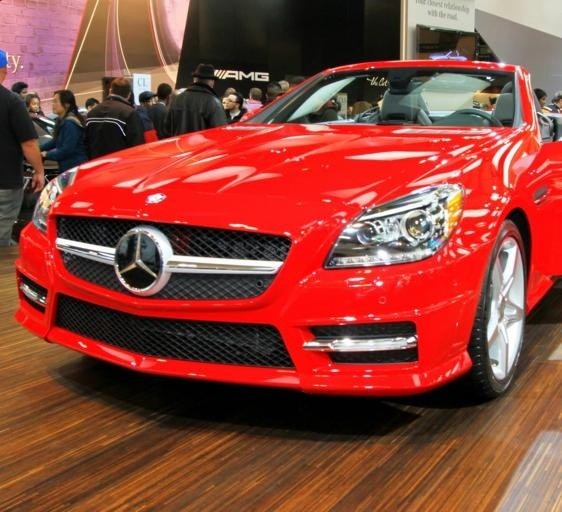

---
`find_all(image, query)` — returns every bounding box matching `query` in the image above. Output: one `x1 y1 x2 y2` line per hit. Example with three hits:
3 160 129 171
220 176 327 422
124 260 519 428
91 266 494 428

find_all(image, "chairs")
485 77 557 141
362 77 432 127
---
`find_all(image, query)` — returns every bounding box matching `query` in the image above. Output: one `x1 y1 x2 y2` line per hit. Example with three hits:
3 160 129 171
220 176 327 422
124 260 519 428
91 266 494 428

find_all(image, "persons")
1 51 45 247
534 88 561 113
11 77 148 174
306 96 377 124
129 62 290 144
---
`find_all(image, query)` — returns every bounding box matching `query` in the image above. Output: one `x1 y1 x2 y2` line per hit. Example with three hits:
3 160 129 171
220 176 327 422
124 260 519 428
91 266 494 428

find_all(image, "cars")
23 112 59 170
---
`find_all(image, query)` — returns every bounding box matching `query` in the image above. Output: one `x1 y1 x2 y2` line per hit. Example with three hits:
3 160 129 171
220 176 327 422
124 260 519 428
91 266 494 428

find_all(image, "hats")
192 63 219 80
139 91 157 102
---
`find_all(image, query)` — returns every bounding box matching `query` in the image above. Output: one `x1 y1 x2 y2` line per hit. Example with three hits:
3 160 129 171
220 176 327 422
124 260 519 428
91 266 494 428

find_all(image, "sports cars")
13 60 562 404
